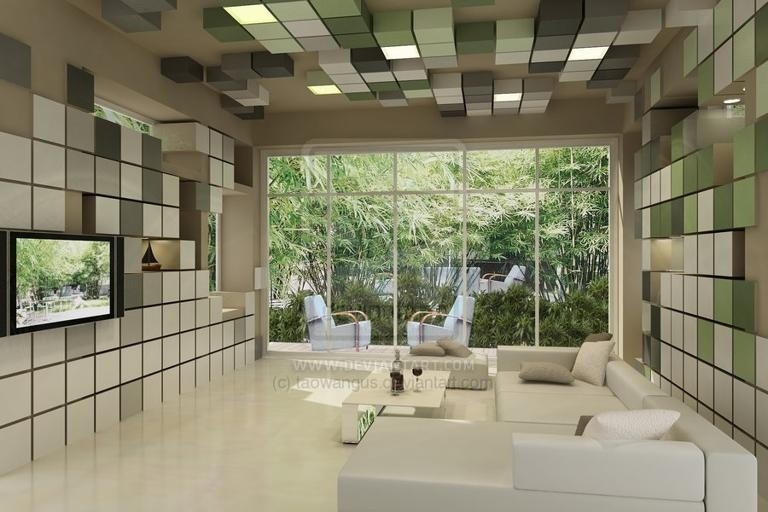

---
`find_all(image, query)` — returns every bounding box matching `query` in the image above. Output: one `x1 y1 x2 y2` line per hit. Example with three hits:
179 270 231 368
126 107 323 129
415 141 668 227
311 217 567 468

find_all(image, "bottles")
389 349 405 393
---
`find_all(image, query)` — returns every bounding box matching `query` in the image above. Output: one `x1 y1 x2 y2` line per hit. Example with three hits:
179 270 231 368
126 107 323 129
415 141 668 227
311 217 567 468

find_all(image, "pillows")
409 336 472 357
574 408 681 440
519 333 615 386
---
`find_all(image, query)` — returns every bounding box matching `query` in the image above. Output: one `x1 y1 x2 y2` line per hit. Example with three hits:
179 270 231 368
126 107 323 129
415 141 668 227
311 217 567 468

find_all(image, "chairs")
407 295 475 348
303 293 371 351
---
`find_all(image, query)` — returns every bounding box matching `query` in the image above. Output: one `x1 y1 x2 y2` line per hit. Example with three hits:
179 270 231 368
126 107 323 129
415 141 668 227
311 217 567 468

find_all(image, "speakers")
0 231 7 337
117 237 124 318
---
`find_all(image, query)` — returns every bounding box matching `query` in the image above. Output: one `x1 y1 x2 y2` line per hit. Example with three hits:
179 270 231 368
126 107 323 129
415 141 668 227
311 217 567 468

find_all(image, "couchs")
338 344 758 512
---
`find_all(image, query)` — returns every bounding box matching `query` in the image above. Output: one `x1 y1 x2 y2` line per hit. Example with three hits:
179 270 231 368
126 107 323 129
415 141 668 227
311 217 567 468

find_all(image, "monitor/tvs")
10 230 114 336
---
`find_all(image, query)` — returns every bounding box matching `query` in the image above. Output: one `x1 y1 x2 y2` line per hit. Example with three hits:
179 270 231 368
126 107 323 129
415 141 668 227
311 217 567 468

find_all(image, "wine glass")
412 363 423 394
389 367 401 396
